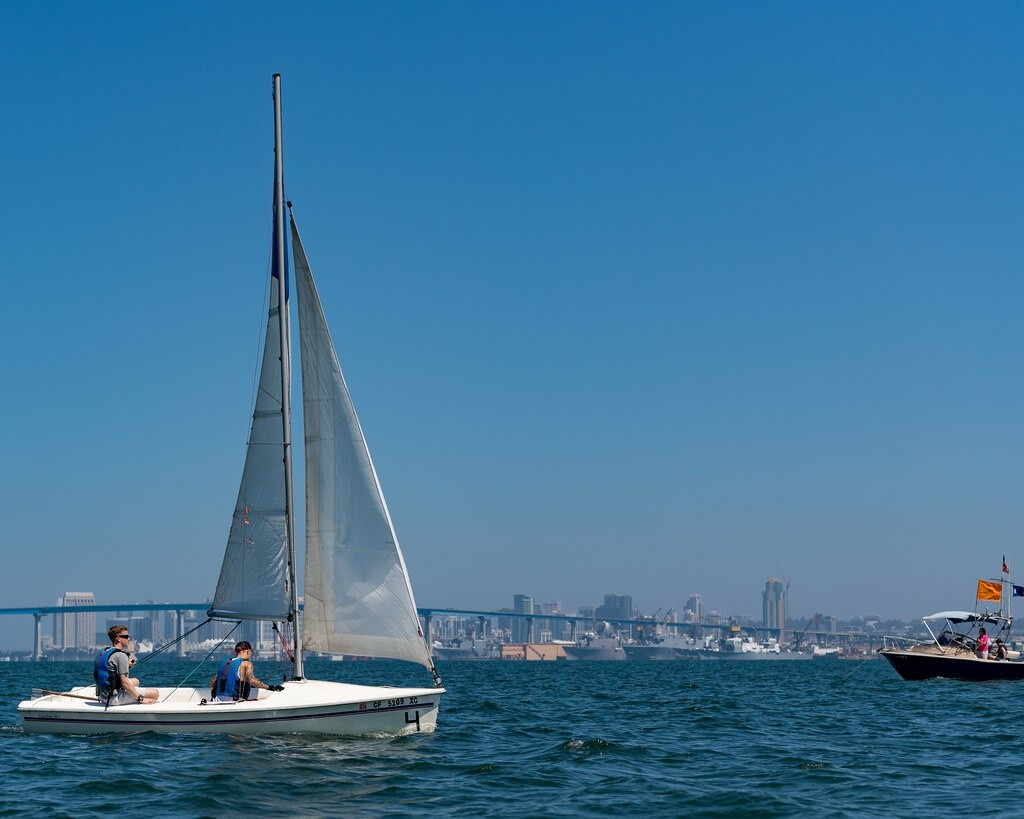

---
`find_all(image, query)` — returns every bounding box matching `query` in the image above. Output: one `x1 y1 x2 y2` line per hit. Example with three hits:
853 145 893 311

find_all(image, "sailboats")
16 71 446 738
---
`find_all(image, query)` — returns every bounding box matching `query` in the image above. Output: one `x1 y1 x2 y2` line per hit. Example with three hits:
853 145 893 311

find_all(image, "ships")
561 623 816 662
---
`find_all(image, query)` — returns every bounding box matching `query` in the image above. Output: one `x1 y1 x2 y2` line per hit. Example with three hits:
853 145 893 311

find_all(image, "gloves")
267 685 284 691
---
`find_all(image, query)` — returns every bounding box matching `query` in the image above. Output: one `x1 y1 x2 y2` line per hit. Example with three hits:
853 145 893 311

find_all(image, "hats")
996 639 1003 643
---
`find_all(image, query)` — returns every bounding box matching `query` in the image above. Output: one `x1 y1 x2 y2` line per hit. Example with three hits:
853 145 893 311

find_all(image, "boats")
880 554 1024 681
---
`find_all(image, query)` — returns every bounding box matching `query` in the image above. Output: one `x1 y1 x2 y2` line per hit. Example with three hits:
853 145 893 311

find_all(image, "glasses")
119 635 129 638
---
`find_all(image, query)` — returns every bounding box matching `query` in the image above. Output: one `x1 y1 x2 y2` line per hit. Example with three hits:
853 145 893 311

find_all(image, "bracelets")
137 695 144 702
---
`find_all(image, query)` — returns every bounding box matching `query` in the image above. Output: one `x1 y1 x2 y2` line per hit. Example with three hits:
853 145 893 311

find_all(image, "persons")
987 639 1007 661
93 625 159 706
209 641 285 703
975 628 988 660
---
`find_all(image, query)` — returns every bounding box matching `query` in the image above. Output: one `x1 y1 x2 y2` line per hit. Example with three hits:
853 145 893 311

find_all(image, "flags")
976 580 1003 602
1003 555 1009 574
1012 586 1024 597
